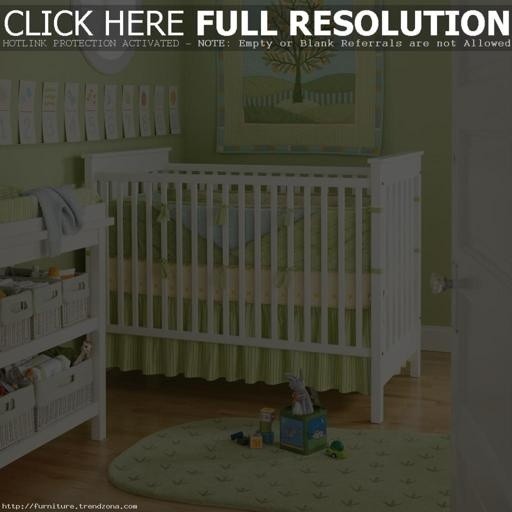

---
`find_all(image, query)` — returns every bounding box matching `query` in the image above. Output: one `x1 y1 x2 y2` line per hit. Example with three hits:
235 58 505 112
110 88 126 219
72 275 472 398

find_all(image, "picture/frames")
223 0 377 145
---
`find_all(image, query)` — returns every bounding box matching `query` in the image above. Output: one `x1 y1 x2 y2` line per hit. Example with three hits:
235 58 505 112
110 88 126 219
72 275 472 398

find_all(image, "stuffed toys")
230 369 351 461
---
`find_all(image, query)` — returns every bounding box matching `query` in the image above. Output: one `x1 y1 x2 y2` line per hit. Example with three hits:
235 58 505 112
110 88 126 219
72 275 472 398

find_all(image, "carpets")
108 415 451 512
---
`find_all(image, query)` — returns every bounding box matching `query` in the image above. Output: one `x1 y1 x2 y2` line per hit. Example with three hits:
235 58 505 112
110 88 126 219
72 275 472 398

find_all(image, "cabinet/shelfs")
0 204 115 469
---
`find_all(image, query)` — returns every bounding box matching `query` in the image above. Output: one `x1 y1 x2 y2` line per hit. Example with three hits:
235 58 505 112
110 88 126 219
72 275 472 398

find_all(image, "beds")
81 146 425 423
